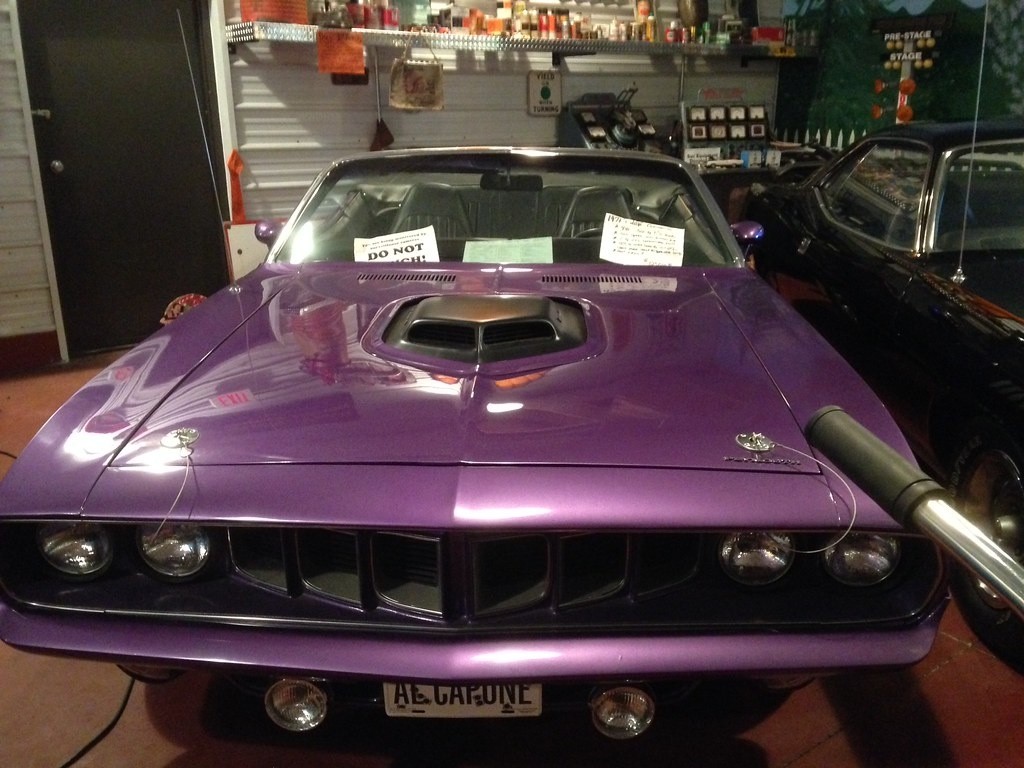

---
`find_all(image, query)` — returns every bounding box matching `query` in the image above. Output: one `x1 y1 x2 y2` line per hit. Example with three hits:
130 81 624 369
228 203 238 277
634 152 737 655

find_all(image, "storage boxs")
750 27 786 46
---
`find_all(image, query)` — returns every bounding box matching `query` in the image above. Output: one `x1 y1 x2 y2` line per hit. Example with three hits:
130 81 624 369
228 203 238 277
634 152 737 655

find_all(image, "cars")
735 115 1024 676
2 137 1023 768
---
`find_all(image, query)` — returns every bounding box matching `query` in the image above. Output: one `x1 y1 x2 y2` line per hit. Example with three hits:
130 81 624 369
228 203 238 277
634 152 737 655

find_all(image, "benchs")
460 185 575 238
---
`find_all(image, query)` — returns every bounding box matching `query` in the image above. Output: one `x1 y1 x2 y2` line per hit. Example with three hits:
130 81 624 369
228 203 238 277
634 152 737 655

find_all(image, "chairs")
885 178 977 243
556 185 635 237
386 182 474 238
